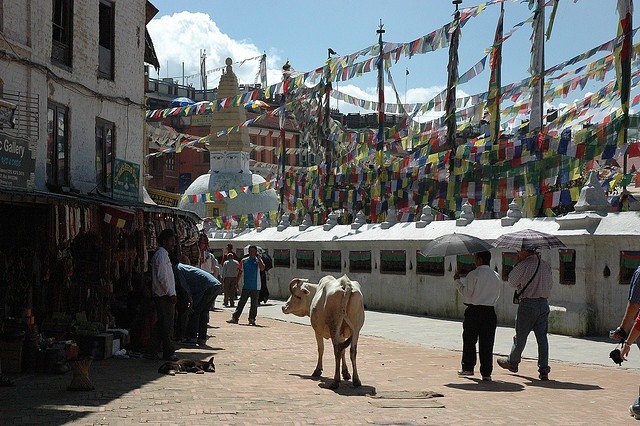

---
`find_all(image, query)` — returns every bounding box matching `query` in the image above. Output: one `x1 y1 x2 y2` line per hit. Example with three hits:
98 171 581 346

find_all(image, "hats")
157 228 179 241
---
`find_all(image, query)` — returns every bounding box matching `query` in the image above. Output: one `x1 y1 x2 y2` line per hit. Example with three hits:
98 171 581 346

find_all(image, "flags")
144 2 640 227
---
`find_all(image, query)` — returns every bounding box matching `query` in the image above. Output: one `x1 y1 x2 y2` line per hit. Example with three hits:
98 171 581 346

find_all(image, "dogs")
157 357 215 376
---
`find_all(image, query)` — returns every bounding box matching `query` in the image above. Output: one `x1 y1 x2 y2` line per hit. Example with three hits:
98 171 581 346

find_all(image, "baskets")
67 357 93 391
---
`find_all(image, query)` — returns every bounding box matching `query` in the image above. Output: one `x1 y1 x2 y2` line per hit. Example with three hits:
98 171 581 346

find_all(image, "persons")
222 253 241 307
454 251 502 383
620 311 640 420
198 228 217 275
612 265 640 342
166 257 222 345
258 250 269 307
226 246 265 326
222 244 238 265
496 248 553 382
140 228 181 362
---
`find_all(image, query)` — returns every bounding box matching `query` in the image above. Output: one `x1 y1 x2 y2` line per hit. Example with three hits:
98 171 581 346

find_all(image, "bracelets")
624 340 631 346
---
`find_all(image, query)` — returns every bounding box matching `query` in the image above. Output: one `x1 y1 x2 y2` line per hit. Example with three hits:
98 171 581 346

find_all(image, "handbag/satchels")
512 290 522 304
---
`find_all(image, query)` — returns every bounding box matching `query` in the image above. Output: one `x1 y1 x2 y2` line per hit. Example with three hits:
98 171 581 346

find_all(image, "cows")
281 273 365 390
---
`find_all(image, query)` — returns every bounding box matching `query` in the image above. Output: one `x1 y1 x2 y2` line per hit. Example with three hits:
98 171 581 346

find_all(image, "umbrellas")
490 229 565 252
416 233 493 270
244 245 265 256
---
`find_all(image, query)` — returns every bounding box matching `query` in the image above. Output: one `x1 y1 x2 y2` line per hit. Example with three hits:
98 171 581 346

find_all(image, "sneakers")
497 358 519 373
483 377 492 383
539 374 549 381
458 370 475 376
226 318 238 323
248 318 255 325
628 405 639 421
